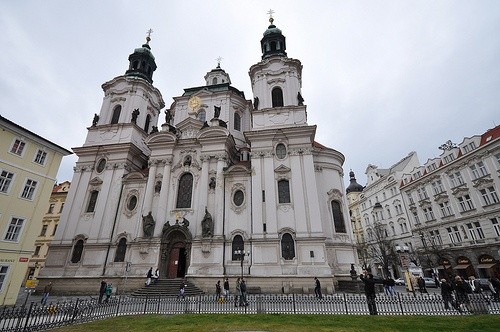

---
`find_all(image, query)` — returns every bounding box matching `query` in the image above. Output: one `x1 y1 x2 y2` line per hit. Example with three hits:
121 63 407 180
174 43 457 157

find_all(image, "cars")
396 277 437 287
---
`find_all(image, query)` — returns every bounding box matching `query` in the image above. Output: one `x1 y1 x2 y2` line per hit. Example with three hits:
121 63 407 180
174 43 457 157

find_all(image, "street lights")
234 250 250 278
396 243 417 297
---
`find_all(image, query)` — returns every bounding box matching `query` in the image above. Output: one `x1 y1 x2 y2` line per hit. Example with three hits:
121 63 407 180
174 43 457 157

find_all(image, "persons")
98 279 113 304
314 277 322 299
224 279 230 297
383 276 397 296
176 283 185 300
233 277 250 307
144 266 153 286
416 277 429 295
440 276 489 312
360 273 378 315
216 280 222 296
41 281 52 306
153 268 160 282
